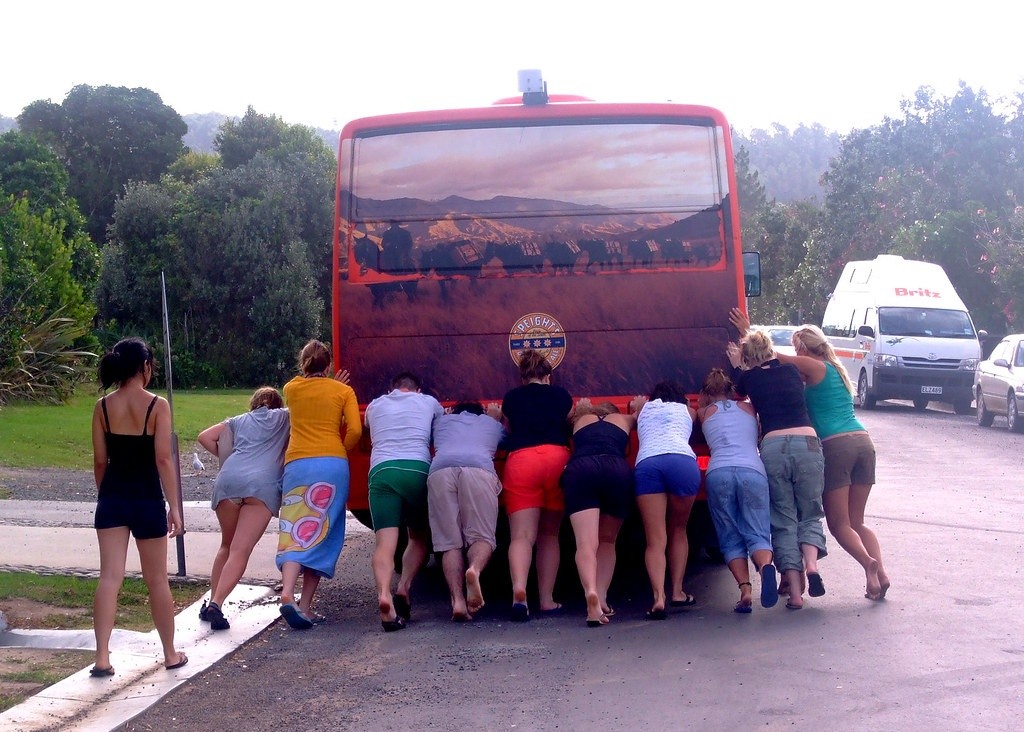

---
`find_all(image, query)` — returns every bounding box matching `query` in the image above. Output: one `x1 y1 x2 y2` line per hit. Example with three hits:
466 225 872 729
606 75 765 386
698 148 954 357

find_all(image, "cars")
971 333 1024 432
763 324 801 356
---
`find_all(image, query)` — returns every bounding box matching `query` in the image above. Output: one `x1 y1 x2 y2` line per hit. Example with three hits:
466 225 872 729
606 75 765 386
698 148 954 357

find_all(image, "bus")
330 68 761 568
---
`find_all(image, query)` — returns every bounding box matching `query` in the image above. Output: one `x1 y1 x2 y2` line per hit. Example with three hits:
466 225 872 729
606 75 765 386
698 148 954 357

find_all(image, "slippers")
603 605 616 617
166 652 188 669
382 615 406 631
646 608 666 620
90 666 114 675
512 603 533 622
808 572 825 597
586 613 610 627
733 601 752 613
307 611 324 623
393 594 411 621
280 604 313 629
761 565 778 608
786 596 803 609
542 603 567 616
670 593 697 606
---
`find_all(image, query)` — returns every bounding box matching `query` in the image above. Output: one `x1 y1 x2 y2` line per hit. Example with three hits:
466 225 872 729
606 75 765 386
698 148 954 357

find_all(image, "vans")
821 254 988 416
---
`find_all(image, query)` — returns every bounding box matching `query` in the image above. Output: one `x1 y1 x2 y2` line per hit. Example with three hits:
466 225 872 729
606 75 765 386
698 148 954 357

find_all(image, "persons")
632 382 703 619
275 341 362 629
195 369 350 630
426 399 510 625
500 348 575 623
726 329 828 610
560 396 637 626
728 307 889 601
89 337 188 677
360 371 446 632
695 367 781 613
380 217 411 262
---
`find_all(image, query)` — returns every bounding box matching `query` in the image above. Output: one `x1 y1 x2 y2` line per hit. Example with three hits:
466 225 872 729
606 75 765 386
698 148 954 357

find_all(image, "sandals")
199 600 208 620
206 602 230 630
